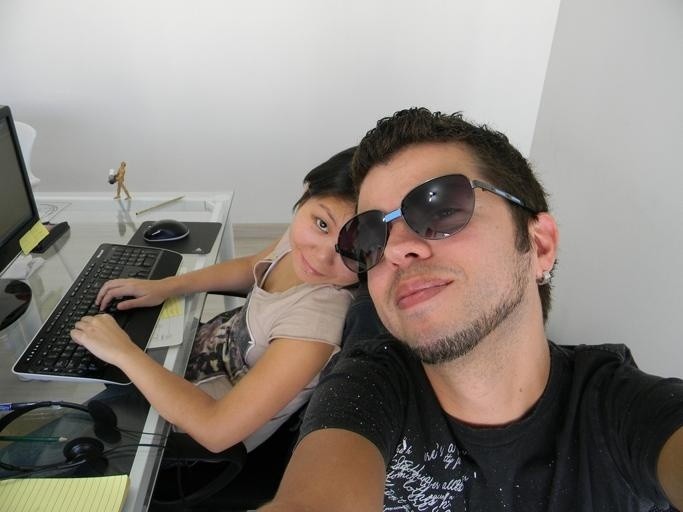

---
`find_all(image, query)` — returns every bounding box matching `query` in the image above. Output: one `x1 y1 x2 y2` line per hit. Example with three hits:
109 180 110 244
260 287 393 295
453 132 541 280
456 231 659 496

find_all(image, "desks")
0 190 239 511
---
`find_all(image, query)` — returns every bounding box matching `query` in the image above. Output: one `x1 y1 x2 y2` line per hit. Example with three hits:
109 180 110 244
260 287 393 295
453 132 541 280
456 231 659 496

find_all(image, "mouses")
143 220 190 243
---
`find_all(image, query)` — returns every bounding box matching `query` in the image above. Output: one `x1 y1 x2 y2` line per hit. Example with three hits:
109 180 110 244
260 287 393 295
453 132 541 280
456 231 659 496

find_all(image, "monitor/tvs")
0 106 40 331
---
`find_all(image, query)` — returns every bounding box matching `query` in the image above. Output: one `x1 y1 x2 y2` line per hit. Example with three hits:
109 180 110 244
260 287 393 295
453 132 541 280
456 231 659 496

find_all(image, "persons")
243 106 683 512
108 159 132 200
70 142 368 510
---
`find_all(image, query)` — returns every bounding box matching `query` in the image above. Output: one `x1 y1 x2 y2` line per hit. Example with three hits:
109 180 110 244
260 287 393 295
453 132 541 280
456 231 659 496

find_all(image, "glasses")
336 173 537 274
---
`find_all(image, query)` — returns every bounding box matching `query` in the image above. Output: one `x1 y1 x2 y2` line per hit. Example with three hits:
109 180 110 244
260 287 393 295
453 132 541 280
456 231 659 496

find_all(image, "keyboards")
11 243 183 386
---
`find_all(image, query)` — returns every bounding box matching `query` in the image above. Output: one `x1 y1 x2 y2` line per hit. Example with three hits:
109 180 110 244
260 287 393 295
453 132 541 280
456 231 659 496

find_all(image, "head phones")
0 400 121 473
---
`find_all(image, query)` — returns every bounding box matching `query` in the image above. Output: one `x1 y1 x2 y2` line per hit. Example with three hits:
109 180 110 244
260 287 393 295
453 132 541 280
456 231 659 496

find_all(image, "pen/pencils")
136 195 185 215
0 400 63 411
0 435 68 442
